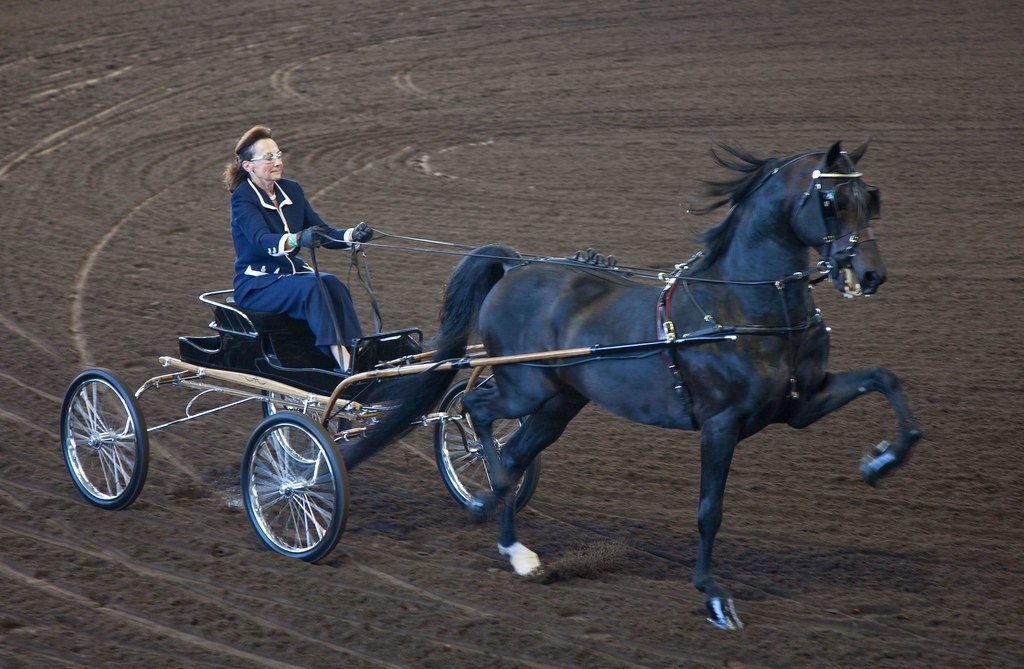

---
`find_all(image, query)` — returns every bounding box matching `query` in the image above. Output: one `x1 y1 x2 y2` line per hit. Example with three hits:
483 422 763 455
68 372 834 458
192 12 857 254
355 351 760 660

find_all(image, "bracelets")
288 234 297 247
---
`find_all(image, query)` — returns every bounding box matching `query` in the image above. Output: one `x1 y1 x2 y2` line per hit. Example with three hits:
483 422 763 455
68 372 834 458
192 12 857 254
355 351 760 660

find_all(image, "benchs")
200 286 313 349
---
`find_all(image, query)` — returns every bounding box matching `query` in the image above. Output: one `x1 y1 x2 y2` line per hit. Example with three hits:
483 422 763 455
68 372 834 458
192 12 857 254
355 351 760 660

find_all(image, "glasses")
249 150 283 161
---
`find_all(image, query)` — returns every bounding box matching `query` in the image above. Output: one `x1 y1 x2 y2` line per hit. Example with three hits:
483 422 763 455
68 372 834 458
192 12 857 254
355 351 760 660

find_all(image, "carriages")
58 134 924 633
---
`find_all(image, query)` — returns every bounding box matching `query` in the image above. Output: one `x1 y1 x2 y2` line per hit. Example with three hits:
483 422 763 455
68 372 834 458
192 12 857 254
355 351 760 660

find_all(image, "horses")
436 136 922 629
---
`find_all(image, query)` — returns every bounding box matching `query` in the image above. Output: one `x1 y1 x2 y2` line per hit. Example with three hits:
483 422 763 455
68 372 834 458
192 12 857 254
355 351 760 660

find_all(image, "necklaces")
268 192 276 200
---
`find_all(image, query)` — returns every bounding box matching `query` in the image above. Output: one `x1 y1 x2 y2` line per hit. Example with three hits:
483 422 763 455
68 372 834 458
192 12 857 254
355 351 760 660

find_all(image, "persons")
225 125 367 376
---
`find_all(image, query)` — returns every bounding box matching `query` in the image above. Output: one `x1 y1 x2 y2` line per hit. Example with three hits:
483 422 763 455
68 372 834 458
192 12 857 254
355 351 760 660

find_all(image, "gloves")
297 225 325 248
352 222 373 243
